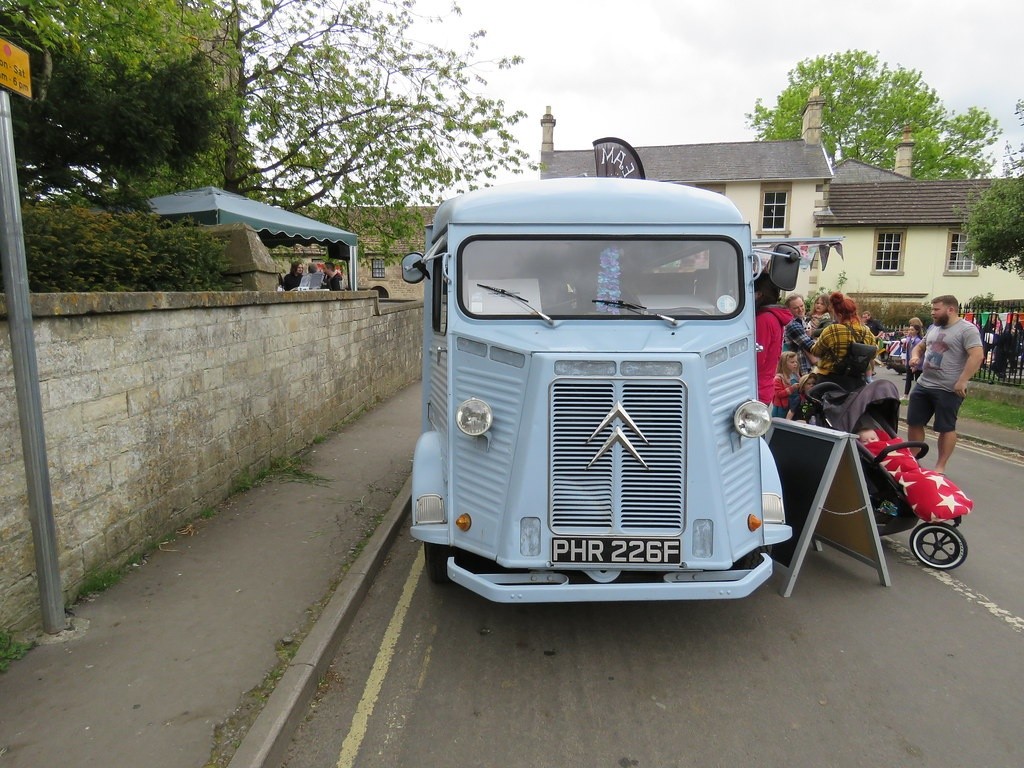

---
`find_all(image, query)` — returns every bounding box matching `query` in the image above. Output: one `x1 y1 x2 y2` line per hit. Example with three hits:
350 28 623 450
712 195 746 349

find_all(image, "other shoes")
872 372 876 376
899 397 909 401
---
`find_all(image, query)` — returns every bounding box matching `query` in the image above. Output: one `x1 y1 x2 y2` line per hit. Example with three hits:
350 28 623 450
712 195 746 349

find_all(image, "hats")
799 373 816 391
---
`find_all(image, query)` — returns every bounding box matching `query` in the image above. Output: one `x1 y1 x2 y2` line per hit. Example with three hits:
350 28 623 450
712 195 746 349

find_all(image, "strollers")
798 380 974 570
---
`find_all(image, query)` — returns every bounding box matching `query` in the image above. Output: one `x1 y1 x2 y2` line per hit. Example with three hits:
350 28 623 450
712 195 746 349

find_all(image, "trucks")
402 176 800 604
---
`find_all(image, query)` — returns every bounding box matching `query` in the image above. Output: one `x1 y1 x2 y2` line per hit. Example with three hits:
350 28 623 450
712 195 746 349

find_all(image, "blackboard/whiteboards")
764 419 882 570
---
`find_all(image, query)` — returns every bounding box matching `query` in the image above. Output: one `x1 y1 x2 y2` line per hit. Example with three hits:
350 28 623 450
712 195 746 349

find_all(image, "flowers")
595 244 622 315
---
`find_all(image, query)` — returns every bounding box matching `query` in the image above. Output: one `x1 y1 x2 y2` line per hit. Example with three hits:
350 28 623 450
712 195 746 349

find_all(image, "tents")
96 185 358 292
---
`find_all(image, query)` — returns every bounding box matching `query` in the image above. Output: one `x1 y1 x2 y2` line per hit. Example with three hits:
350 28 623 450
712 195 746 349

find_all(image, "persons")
900 317 927 401
299 263 323 288
771 290 885 421
322 262 343 291
907 295 984 475
283 261 304 291
857 428 974 524
980 310 1024 380
752 272 794 409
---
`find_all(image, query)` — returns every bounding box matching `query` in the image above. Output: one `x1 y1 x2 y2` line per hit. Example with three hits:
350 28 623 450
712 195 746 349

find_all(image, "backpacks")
827 324 877 391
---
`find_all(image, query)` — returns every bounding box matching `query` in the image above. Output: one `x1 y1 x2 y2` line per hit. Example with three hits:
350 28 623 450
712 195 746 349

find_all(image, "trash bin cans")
345 285 390 299
